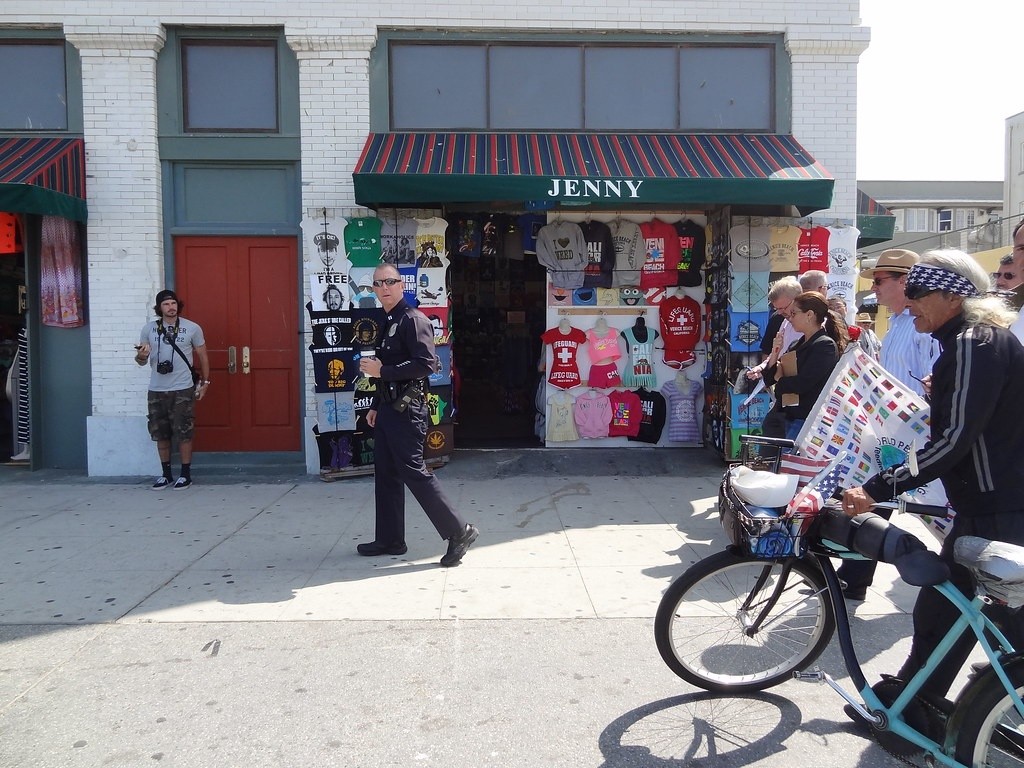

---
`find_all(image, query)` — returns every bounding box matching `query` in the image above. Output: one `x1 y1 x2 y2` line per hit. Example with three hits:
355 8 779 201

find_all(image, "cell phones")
134 346 145 350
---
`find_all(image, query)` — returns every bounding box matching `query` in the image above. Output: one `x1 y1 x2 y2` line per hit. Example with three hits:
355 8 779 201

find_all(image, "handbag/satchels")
192 373 200 391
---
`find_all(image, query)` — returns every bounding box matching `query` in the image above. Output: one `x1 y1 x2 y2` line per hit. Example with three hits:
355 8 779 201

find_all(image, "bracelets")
205 381 210 384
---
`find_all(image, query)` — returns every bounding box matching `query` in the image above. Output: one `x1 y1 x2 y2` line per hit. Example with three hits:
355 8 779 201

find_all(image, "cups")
361 351 376 377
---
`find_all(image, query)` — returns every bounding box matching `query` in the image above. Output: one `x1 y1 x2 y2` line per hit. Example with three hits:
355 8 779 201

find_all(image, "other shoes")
970 662 990 674
802 572 866 600
844 704 871 728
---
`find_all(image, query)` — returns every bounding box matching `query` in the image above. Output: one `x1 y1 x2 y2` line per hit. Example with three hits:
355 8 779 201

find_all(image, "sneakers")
440 523 480 566
173 474 193 491
357 540 407 556
152 476 175 491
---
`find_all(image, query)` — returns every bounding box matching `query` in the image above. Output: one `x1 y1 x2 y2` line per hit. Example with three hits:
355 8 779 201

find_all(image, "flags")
802 348 956 547
780 452 842 536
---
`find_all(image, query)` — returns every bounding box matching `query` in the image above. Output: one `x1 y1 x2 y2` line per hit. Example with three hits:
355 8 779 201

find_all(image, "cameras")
157 360 173 375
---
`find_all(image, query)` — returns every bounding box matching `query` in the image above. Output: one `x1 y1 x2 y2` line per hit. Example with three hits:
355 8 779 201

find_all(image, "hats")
855 313 875 325
156 290 177 305
313 232 339 250
860 249 920 279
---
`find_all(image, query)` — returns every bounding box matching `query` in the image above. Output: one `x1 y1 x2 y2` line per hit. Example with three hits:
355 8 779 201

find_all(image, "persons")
657 289 702 369
1007 218 1024 346
323 284 344 311
583 318 622 389
855 312 882 361
803 249 943 599
314 232 339 266
841 248 1024 727
135 290 209 490
620 317 660 387
383 238 410 263
540 318 586 389
992 252 1021 292
356 263 480 566
661 369 704 442
746 270 876 458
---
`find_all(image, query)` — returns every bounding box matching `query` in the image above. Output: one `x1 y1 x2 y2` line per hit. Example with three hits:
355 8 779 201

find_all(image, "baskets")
718 462 822 561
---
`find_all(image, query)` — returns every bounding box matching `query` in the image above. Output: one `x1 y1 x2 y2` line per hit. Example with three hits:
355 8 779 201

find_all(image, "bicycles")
654 485 1024 768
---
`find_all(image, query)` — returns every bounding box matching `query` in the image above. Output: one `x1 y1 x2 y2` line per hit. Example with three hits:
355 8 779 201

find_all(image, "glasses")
773 301 792 311
872 273 907 285
828 305 846 311
993 272 1017 279
373 278 402 287
791 309 807 318
905 284 942 300
819 285 831 290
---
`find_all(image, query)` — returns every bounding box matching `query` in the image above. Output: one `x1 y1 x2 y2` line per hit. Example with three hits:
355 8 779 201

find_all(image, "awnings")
0 138 88 224
857 189 896 249
352 133 835 217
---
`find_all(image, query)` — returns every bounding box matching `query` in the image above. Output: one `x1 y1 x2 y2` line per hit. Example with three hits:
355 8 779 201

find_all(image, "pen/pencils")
744 366 757 376
776 328 785 353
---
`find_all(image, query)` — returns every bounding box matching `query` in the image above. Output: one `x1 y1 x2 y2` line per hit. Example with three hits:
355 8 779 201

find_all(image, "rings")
847 504 854 508
